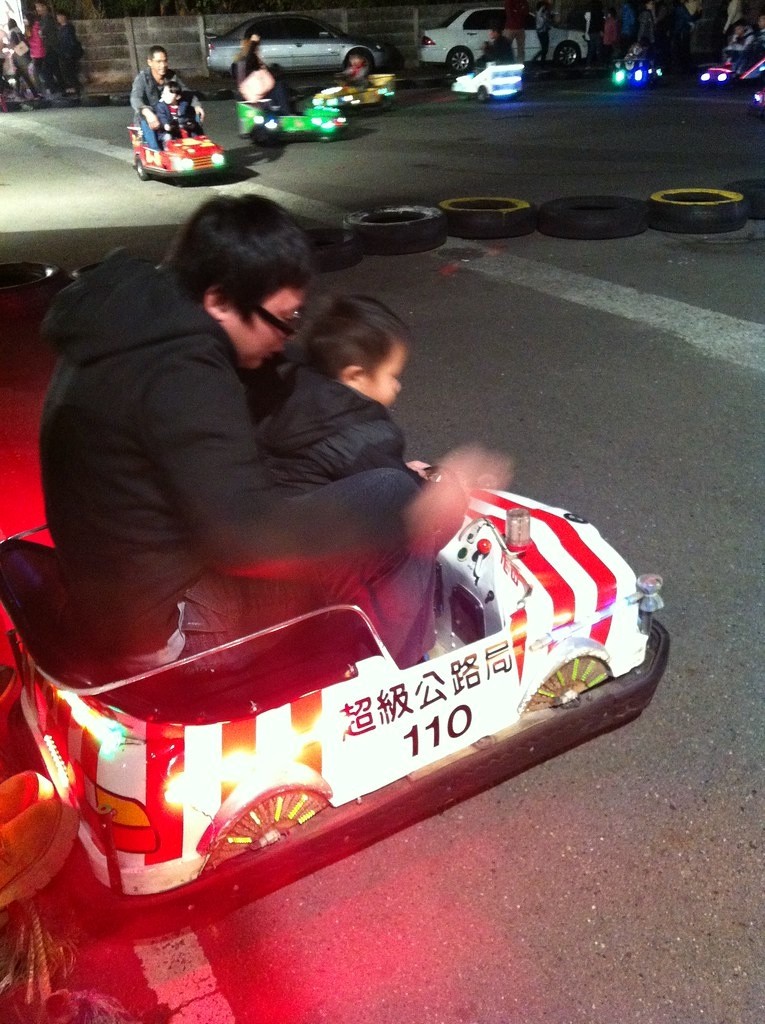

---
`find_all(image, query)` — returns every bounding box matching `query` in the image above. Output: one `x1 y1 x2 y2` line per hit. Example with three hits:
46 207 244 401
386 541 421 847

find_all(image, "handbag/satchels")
12 31 29 57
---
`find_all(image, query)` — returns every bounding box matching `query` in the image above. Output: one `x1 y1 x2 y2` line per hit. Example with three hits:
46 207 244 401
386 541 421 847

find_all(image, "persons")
339 52 373 89
531 0 551 68
242 32 297 116
30 193 505 670
261 295 429 511
0 3 81 111
482 26 514 64
502 0 526 62
129 45 206 148
577 0 765 76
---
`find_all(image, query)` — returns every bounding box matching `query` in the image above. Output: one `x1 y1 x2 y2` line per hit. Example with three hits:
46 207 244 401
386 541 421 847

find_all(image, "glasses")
258 303 305 342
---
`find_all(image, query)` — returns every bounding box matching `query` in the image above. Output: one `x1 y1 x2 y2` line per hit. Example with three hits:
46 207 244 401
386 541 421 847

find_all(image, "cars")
417 6 590 76
204 12 391 81
